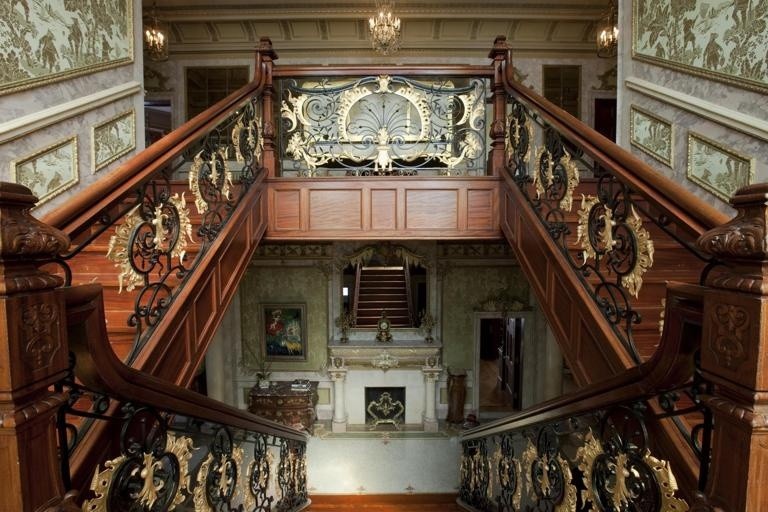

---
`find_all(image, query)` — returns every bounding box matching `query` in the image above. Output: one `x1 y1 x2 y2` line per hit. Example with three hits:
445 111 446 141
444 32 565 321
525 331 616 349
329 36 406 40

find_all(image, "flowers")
419 309 438 328
335 308 355 329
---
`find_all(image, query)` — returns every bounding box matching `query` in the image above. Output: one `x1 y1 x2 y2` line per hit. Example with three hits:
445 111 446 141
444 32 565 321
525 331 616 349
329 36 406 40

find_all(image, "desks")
246 381 319 436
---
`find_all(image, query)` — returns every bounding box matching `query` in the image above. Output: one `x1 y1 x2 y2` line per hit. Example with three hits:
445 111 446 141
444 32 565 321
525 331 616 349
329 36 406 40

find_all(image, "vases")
424 327 433 344
445 373 468 422
341 327 348 343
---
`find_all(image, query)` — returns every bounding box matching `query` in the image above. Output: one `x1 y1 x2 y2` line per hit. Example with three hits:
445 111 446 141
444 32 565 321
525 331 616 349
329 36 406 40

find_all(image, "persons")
268 310 286 336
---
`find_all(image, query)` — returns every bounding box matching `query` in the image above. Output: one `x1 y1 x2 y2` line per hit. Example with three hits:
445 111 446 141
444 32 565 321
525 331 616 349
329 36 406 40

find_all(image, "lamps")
142 0 169 62
368 0 401 56
591 0 619 58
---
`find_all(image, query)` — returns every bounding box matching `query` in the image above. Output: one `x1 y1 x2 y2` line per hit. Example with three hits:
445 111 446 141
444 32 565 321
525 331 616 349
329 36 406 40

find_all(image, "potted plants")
256 363 271 389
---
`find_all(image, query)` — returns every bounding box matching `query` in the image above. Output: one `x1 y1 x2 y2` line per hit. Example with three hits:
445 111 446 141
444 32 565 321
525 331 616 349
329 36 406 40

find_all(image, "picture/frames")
256 302 308 362
337 242 434 330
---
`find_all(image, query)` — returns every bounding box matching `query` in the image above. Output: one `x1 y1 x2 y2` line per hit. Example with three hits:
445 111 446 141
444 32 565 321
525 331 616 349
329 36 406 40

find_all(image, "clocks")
376 311 392 342
